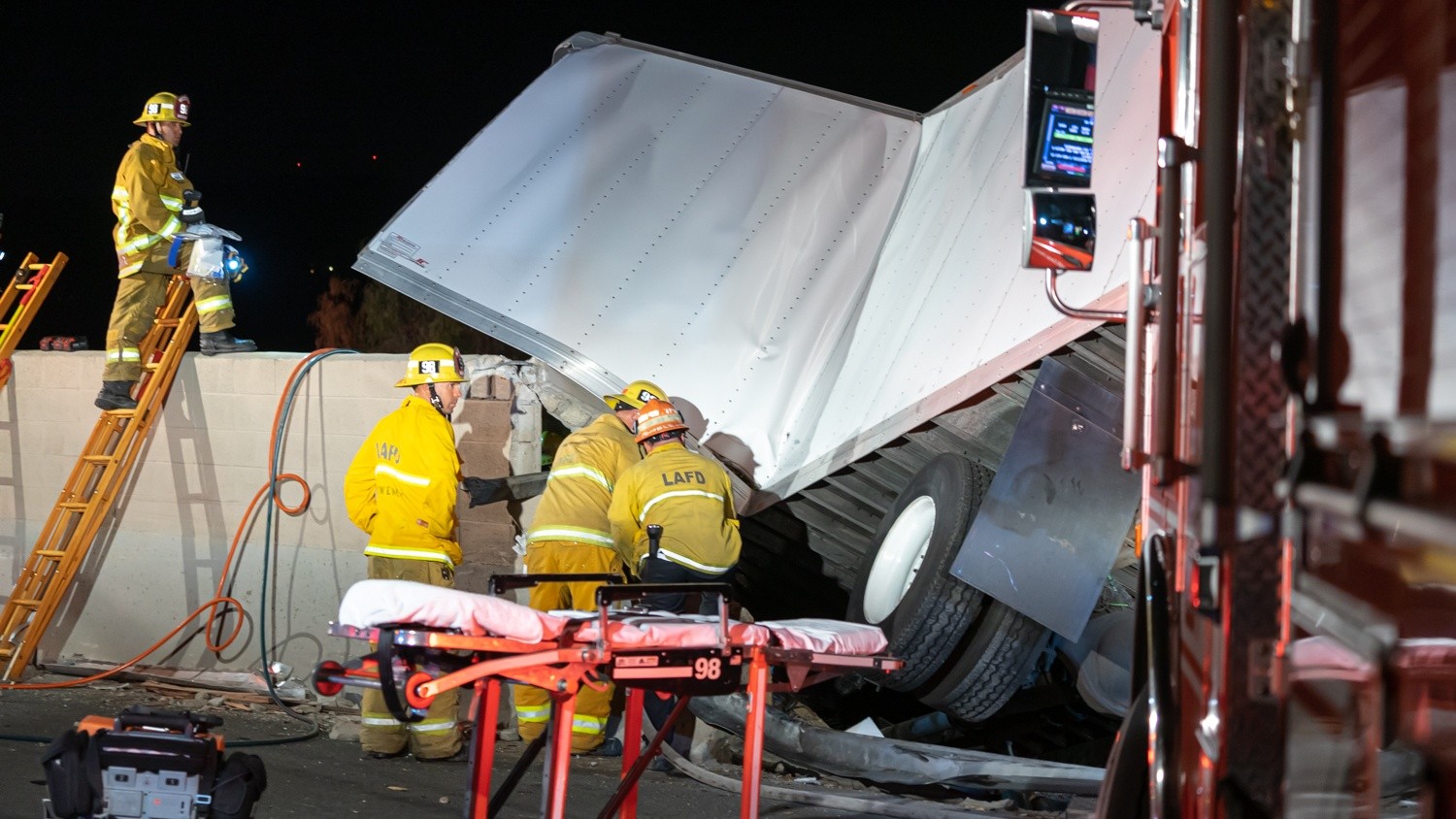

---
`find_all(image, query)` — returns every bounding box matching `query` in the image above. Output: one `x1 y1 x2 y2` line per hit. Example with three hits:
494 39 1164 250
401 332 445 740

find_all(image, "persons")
608 399 743 770
516 380 684 757
94 92 257 412
344 341 471 762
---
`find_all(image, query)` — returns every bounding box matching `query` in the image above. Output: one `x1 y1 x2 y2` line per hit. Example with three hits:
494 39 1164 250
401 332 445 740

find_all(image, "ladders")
0 250 68 365
0 273 199 683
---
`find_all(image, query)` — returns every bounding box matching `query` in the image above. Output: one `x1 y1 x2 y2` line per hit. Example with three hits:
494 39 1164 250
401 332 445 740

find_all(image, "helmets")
603 382 670 411
635 398 690 441
133 91 189 128
394 342 470 387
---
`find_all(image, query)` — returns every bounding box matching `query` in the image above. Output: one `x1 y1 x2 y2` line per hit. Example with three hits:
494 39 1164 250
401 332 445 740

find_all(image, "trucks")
347 26 1139 819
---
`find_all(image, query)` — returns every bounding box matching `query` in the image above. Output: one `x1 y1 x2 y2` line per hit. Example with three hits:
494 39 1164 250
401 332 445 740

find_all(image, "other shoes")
419 746 470 762
641 744 677 772
570 737 621 758
362 740 412 758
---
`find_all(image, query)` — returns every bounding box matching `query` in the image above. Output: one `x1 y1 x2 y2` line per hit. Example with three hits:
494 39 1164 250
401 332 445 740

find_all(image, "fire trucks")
1014 0 1456 819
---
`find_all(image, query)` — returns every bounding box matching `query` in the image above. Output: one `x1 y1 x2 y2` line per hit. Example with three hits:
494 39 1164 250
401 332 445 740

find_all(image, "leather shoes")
201 330 257 357
94 381 151 421
